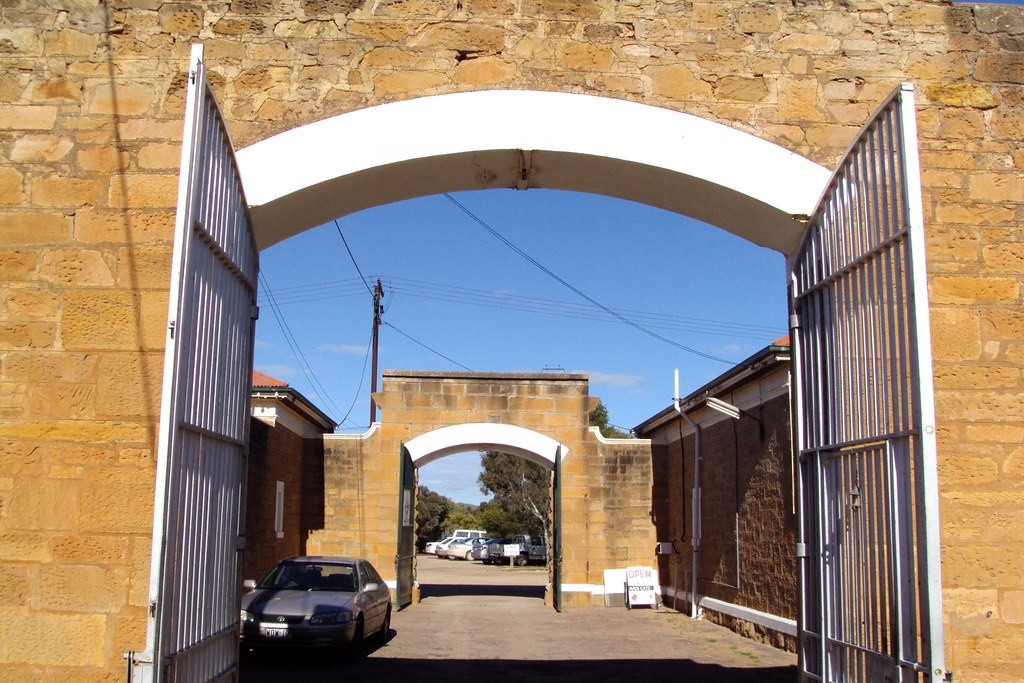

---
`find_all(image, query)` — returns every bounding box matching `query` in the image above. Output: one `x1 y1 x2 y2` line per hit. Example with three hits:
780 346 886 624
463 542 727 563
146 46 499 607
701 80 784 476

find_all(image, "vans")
453 530 486 538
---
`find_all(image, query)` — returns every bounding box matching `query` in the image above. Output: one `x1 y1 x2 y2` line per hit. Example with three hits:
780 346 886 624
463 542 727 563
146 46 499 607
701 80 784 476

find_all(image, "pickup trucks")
500 534 545 565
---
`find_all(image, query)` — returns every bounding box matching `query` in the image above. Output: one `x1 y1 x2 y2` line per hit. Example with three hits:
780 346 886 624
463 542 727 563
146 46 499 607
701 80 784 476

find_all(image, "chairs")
328 574 353 592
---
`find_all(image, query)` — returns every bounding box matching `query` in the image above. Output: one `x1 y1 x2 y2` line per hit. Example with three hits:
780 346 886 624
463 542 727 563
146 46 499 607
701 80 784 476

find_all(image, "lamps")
706 398 768 446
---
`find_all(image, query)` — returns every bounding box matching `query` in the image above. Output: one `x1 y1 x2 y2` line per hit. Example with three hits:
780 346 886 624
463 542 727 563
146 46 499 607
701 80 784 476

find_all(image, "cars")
240 555 392 666
425 536 513 565
